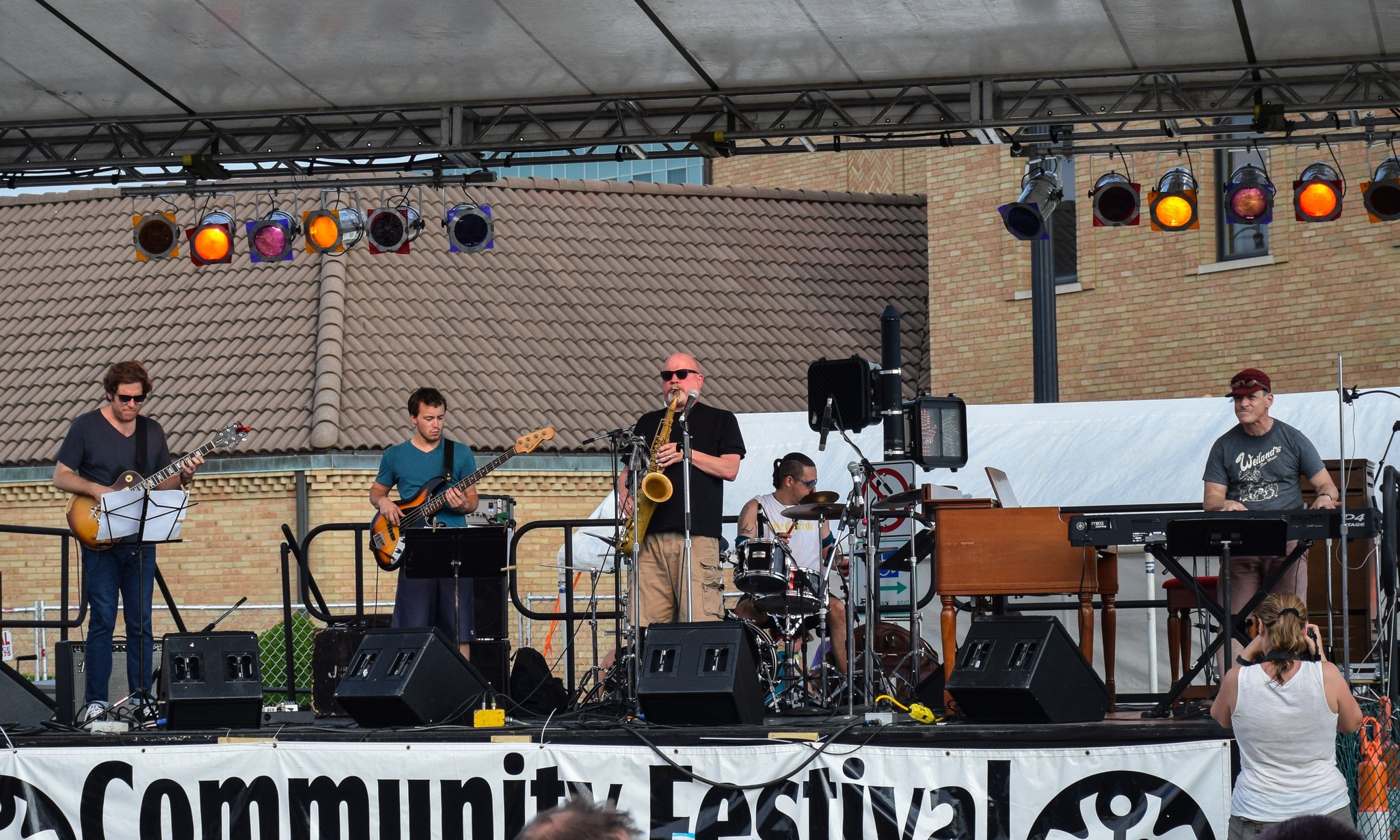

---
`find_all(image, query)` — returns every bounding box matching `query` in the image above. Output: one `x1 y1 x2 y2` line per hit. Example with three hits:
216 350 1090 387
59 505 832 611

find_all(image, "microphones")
847 460 865 510
679 389 700 424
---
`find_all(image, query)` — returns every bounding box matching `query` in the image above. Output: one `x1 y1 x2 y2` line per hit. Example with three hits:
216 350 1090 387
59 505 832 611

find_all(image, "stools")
1161 576 1236 715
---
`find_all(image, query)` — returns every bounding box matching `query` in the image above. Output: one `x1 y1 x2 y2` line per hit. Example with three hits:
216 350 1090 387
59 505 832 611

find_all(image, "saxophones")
615 386 680 557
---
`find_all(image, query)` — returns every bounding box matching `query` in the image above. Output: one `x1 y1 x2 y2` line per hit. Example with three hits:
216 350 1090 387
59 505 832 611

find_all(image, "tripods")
76 484 200 737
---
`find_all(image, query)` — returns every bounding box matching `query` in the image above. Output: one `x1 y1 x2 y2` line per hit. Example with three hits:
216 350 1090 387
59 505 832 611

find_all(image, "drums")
733 538 792 596
751 566 831 620
723 615 779 699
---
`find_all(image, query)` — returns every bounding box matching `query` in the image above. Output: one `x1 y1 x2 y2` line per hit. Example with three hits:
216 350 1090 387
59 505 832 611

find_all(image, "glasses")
660 369 698 381
1229 378 1270 393
783 474 818 488
113 389 147 404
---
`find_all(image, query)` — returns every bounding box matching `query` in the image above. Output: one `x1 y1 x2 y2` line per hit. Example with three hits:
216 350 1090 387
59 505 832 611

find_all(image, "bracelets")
1317 494 1332 501
180 471 191 485
1236 655 1254 667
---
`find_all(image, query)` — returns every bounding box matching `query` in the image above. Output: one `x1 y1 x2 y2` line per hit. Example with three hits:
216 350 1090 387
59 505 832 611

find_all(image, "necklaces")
772 493 799 529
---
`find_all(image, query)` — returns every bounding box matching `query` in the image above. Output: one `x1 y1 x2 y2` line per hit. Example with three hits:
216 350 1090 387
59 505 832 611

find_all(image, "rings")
668 455 672 460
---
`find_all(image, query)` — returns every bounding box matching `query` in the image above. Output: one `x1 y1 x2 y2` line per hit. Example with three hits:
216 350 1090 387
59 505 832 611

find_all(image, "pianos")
1067 506 1383 719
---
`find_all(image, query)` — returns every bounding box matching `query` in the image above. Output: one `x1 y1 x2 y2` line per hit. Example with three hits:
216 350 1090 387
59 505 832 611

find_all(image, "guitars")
368 420 555 572
65 420 251 552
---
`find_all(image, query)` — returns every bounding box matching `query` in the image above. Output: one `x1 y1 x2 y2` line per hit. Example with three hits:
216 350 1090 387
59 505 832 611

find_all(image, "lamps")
993 138 1400 242
119 165 500 268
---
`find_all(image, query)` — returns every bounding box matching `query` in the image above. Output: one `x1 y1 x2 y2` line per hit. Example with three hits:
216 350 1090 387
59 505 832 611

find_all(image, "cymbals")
800 491 840 505
780 502 862 521
870 485 958 509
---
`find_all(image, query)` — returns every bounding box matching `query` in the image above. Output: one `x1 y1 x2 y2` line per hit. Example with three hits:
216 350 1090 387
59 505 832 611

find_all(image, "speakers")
157 630 263 730
945 615 1115 722
638 621 767 726
333 625 506 729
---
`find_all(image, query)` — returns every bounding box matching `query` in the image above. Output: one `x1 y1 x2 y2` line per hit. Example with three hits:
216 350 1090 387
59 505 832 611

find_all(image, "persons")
734 453 856 706
53 360 205 730
617 353 747 626
1211 591 1363 840
1255 814 1364 840
588 643 636 703
1201 368 1342 686
369 387 479 661
513 801 631 840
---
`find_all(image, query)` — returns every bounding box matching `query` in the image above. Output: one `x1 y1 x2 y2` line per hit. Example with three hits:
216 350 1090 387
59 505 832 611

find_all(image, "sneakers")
133 706 159 728
83 705 108 729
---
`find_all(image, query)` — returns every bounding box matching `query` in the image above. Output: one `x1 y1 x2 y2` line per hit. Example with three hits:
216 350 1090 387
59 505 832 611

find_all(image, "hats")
1225 369 1271 397
